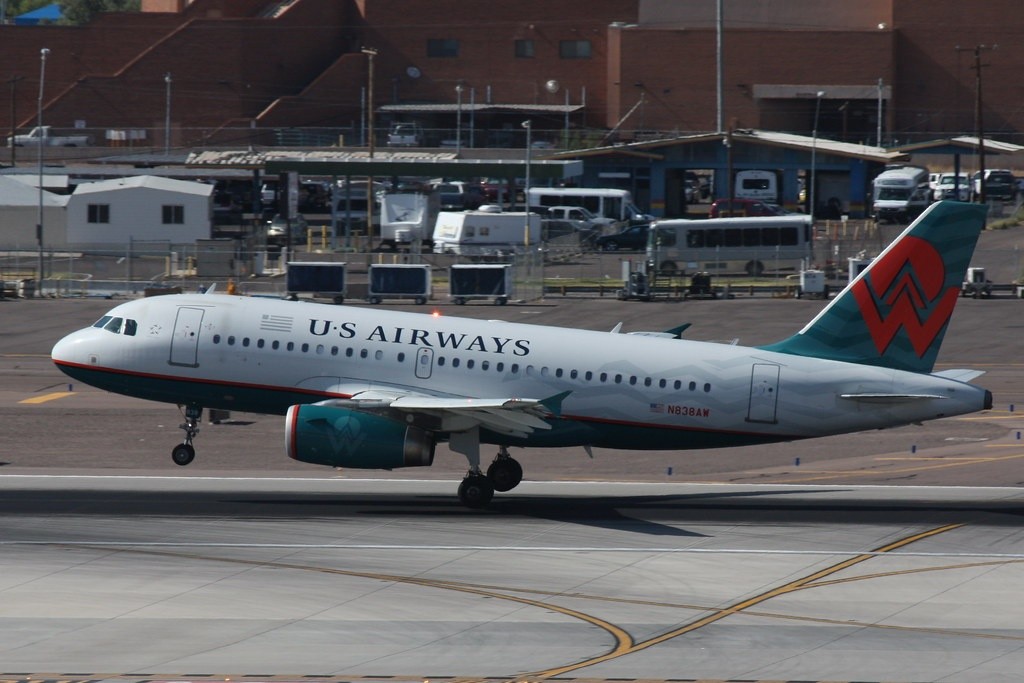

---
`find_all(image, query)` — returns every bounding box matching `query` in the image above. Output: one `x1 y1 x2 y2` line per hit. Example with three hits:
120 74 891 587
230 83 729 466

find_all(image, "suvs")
508 203 648 252
928 171 972 203
707 198 794 218
973 168 1019 203
210 178 512 226
386 121 428 147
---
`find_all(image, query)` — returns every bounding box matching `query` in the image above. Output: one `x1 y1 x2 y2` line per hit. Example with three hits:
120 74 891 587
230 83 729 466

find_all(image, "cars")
267 213 309 246
685 178 701 204
440 132 471 149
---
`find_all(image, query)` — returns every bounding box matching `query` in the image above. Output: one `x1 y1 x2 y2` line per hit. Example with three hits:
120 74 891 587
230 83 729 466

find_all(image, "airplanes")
50 201 994 509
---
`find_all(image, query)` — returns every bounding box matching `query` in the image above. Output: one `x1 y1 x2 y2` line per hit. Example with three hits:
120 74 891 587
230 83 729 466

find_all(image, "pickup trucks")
7 125 93 148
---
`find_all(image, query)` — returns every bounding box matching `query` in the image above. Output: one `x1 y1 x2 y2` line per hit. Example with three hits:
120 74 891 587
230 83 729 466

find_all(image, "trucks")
735 170 777 207
529 187 655 226
865 166 929 224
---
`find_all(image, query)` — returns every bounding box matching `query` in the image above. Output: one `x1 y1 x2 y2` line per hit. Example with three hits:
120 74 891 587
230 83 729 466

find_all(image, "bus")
646 212 812 276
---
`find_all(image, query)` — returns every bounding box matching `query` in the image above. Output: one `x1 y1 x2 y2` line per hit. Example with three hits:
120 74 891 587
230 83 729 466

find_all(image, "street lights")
810 90 827 213
38 47 52 279
164 71 173 160
522 119 532 246
362 50 377 159
